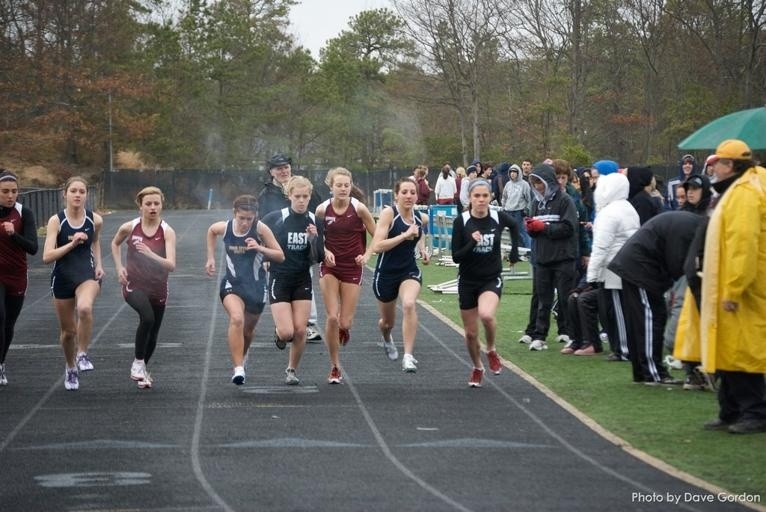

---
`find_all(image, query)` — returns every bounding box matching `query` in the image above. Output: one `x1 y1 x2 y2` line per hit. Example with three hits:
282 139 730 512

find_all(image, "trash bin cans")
433 205 459 250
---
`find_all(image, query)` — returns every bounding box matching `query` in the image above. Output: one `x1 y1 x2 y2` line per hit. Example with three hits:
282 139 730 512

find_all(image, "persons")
42 176 106 391
204 194 286 385
314 167 377 384
408 139 766 434
111 186 177 389
259 175 319 385
256 154 323 341
370 177 430 374
0 168 38 386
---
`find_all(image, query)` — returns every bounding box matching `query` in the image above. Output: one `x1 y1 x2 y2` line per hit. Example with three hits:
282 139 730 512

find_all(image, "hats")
266 155 291 171
682 177 702 189
706 140 751 162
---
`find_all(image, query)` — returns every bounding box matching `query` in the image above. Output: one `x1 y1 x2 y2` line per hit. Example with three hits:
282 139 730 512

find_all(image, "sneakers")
285 367 299 384
339 330 350 346
0 363 8 387
519 332 621 360
275 326 286 350
65 362 79 390
232 367 245 385
130 358 151 389
383 333 400 361
329 367 342 384
469 367 485 387
74 349 93 371
633 366 716 393
402 354 417 373
305 323 323 340
487 350 502 376
704 416 766 435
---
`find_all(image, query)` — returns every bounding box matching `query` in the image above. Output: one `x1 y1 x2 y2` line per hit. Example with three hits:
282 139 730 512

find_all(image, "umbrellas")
677 107 766 151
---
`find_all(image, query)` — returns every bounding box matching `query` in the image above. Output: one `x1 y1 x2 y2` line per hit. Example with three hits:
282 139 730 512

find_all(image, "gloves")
525 216 544 232
